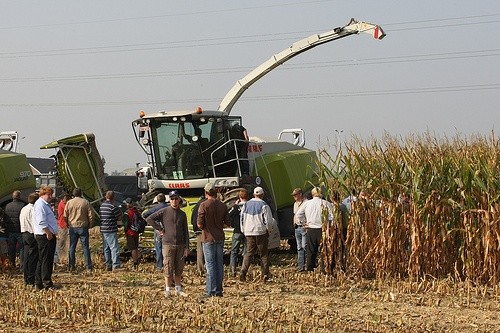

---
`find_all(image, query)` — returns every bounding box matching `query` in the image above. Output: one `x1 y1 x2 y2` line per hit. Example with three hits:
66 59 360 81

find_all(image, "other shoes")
263 274 268 283
70 267 74 271
238 280 246 284
10 269 15 272
175 291 188 298
45 284 61 291
35 284 43 290
86 266 92 269
113 268 126 272
166 291 172 299
105 268 111 270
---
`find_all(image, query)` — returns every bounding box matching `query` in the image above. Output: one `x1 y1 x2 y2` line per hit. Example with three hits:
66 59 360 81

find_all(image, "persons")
237 186 274 281
191 189 216 277
225 188 258 279
181 124 209 150
139 192 170 272
121 197 149 270
143 189 190 298
289 185 367 273
2 185 94 290
197 182 226 298
98 189 124 271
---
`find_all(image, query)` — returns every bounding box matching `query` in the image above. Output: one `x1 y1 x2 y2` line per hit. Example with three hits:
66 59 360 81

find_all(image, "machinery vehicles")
0 130 110 238
130 18 386 258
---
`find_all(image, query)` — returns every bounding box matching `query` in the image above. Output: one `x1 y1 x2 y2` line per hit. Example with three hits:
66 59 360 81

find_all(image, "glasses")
171 198 178 200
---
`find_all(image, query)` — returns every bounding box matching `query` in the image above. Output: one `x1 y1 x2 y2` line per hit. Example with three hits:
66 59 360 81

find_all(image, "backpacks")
131 211 148 233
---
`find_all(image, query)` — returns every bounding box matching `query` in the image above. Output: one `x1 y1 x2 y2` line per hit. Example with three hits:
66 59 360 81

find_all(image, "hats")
169 191 179 197
126 197 132 204
205 183 217 192
290 187 302 196
254 187 264 195
